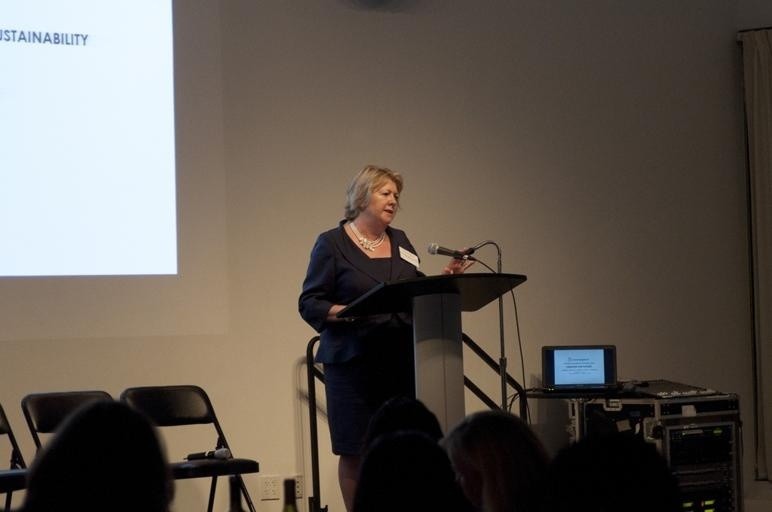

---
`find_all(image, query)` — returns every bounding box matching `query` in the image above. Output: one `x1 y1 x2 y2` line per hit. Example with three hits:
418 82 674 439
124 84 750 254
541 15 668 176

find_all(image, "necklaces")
349 223 387 251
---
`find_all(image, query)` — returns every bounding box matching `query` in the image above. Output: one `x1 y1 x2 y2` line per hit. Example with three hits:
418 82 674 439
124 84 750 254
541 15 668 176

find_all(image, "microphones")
186 448 230 460
428 243 476 262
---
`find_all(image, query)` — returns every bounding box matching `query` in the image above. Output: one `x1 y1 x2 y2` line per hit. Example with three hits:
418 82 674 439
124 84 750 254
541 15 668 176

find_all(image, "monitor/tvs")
541 345 618 391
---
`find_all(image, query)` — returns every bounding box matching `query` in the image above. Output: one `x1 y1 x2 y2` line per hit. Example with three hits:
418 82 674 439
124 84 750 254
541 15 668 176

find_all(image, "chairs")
1 385 259 511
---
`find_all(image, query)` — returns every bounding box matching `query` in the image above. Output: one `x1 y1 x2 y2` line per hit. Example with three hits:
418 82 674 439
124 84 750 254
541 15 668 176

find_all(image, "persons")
16 399 179 512
344 392 685 511
296 163 480 512
